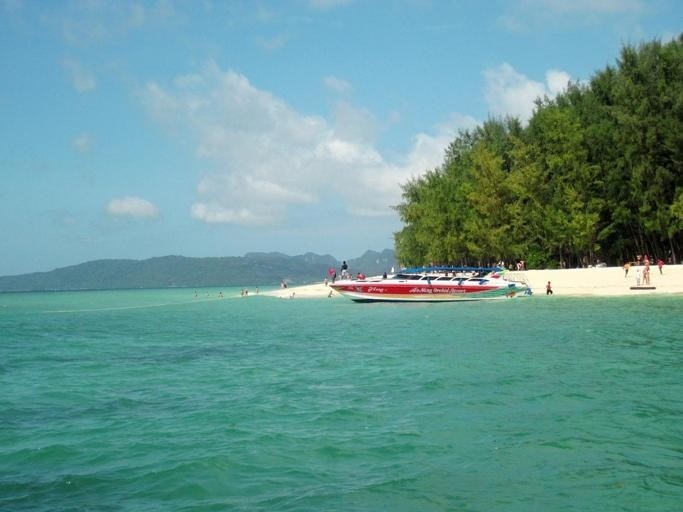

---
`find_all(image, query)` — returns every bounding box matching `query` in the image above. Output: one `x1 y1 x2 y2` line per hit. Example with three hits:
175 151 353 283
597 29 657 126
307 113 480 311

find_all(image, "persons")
624 254 665 287
546 281 554 295
281 279 300 299
425 259 533 278
193 287 259 300
383 271 388 279
323 261 366 300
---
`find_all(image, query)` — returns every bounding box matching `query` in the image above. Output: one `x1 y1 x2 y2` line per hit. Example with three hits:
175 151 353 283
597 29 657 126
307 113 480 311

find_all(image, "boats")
327 272 528 302
